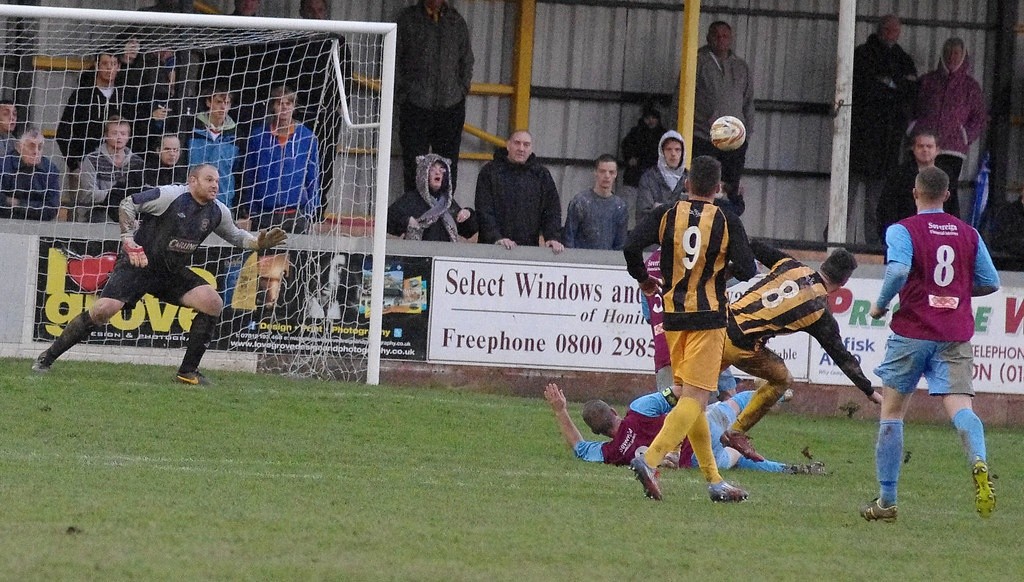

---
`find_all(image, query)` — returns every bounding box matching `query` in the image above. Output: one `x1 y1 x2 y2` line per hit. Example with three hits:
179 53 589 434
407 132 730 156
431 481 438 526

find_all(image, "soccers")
710 116 746 151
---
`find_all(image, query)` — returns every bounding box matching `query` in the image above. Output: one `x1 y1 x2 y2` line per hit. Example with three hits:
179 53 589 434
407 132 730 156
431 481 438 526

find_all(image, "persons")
31 162 289 386
878 126 964 253
545 237 883 470
672 20 754 194
392 1 475 194
1 0 352 234
564 154 628 250
622 155 751 503
861 168 999 524
623 108 668 185
824 14 986 245
387 154 479 242
475 129 565 255
636 130 691 228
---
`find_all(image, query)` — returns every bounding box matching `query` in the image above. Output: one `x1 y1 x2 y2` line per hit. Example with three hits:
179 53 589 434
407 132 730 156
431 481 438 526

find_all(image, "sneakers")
860 495 899 524
629 455 664 503
32 358 52 374
790 461 825 476
720 430 766 463
174 370 219 389
753 377 795 401
709 481 750 504
969 456 997 518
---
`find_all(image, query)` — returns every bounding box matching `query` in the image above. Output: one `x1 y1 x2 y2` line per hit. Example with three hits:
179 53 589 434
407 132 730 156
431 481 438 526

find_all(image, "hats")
640 98 662 120
405 154 463 243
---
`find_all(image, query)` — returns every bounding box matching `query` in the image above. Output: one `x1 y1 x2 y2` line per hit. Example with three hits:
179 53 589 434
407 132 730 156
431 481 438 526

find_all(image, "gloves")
120 232 149 267
257 226 289 250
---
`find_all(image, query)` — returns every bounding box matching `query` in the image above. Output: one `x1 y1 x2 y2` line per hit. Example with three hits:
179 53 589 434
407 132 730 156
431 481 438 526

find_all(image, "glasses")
428 167 447 177
